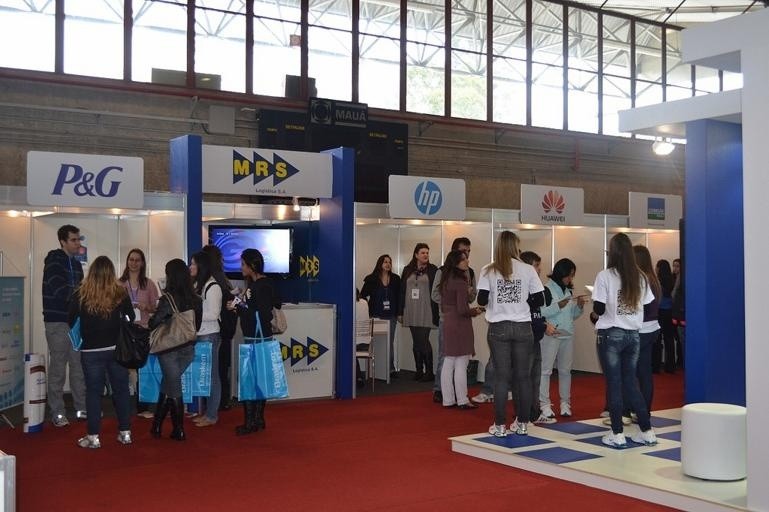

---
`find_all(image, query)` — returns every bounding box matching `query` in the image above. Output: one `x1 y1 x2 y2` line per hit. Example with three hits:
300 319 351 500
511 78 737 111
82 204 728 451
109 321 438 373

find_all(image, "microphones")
680 402 746 481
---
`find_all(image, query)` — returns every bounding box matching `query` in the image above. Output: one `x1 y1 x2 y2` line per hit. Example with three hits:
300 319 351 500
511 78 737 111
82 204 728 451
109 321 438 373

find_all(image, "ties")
207 226 293 279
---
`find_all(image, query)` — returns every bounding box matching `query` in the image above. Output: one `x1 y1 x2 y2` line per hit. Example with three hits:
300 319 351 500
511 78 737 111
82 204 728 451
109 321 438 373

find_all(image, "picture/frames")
373 319 390 384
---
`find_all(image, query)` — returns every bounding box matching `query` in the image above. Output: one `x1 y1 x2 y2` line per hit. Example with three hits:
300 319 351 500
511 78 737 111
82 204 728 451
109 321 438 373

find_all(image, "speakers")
166 394 187 440
254 399 266 431
234 400 260 434
149 391 170 438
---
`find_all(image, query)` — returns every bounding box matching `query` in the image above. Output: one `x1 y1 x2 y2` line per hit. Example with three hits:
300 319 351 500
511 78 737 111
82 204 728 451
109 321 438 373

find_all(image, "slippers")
204 279 238 341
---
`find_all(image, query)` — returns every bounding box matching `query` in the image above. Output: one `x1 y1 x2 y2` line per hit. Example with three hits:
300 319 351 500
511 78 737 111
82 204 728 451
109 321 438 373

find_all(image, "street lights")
235 340 290 402
147 292 198 356
113 318 151 369
134 339 213 405
270 306 287 335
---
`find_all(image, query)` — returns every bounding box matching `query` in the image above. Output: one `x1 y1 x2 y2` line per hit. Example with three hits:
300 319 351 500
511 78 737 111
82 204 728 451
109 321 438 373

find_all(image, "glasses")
652 136 674 155
292 197 300 211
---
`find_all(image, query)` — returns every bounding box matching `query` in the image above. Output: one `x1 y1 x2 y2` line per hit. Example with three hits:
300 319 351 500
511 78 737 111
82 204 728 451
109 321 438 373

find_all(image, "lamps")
355 318 376 393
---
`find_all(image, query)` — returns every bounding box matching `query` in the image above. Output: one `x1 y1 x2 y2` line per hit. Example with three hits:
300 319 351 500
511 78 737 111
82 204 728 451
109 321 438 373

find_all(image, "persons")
431 237 484 409
358 290 370 353
74 255 135 449
228 248 272 435
398 243 439 383
137 258 202 441
115 248 157 417
188 245 239 428
591 234 659 448
472 233 585 437
360 254 404 380
601 246 683 426
44 225 104 427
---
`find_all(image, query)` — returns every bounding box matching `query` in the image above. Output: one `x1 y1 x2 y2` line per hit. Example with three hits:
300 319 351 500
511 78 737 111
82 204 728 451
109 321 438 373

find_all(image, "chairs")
75 409 105 419
488 421 507 438
51 413 70 428
410 371 497 410
560 401 572 417
136 405 218 427
77 433 101 448
600 407 658 449
509 416 528 435
533 415 558 425
540 404 555 417
116 430 132 444
